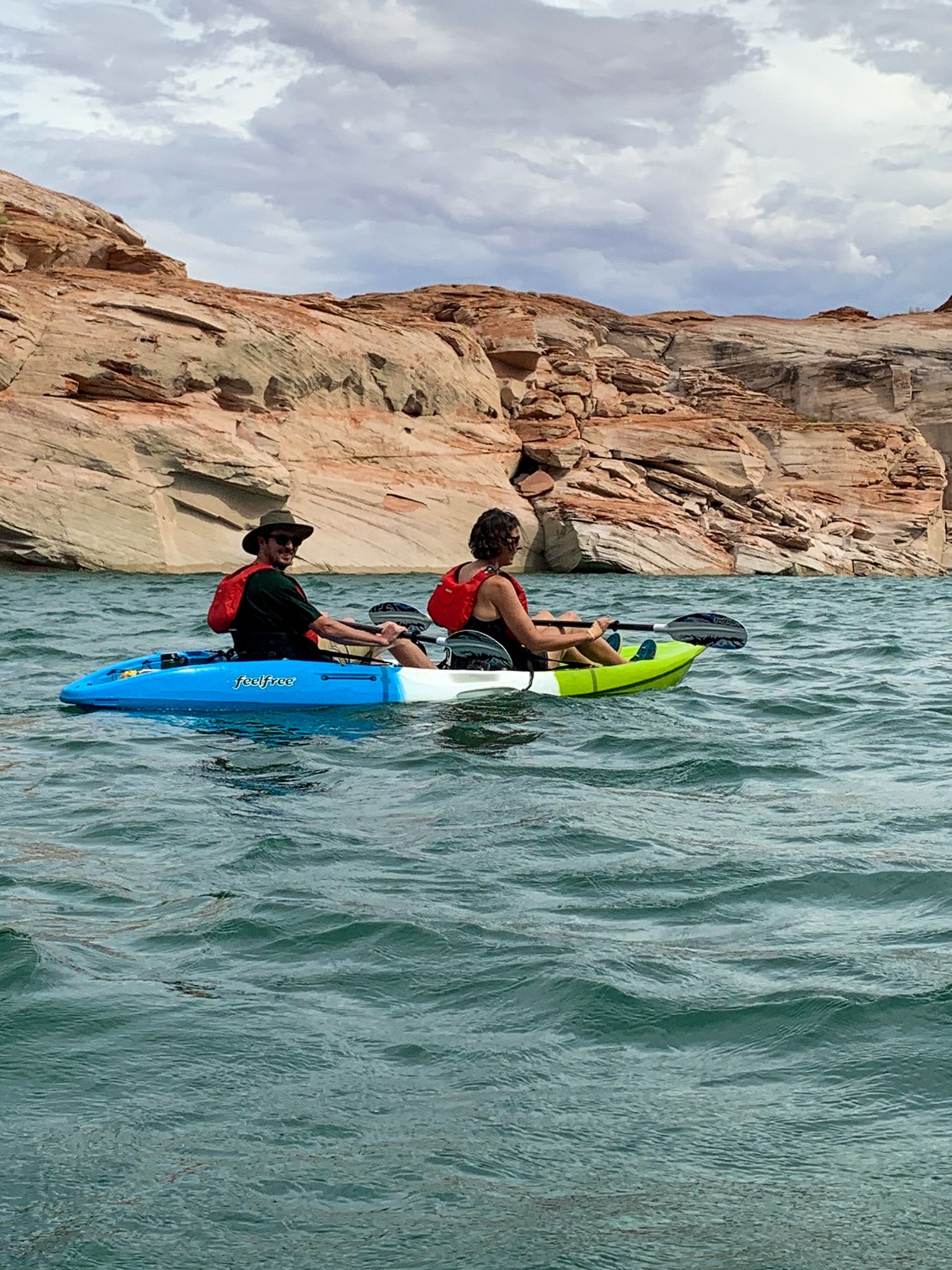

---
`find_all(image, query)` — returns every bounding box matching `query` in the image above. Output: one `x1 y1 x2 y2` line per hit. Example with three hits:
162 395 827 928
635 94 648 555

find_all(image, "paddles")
367 600 749 651
334 617 513 668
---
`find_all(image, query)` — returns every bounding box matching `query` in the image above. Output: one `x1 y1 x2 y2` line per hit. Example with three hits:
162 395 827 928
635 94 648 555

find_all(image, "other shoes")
606 632 622 654
629 640 657 661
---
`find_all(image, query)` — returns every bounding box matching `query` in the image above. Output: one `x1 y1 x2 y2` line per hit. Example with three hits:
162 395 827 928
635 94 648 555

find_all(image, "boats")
58 638 707 713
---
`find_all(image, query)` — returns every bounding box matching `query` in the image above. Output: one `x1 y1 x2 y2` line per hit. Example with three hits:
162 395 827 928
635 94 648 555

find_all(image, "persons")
447 508 656 671
229 509 441 670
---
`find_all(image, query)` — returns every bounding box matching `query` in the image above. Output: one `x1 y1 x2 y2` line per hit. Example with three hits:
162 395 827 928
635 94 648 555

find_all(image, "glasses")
266 534 303 546
506 536 520 544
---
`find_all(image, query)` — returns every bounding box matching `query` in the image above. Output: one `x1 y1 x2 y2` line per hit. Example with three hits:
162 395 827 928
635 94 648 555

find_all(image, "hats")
242 509 314 555
